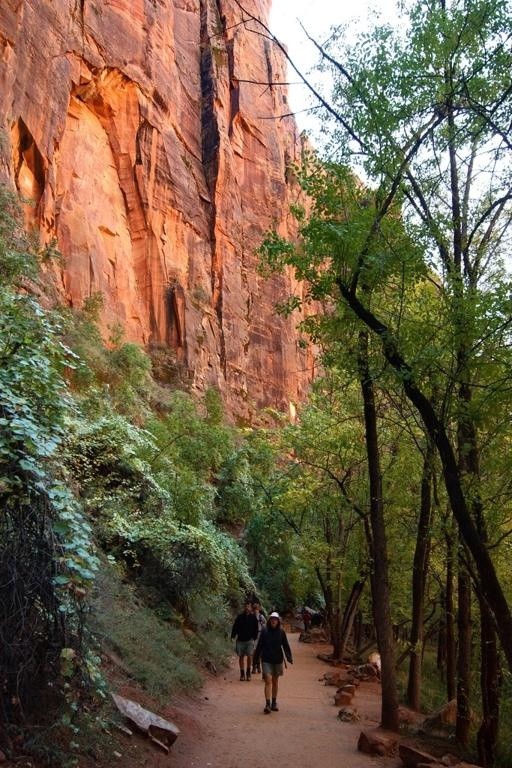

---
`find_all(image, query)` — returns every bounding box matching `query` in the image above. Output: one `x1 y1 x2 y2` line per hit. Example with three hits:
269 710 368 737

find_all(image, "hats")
266 612 283 624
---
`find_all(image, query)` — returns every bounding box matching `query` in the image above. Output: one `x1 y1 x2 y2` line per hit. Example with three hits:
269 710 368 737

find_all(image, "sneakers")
240 674 245 680
272 702 278 710
252 665 261 673
263 705 271 712
247 674 251 681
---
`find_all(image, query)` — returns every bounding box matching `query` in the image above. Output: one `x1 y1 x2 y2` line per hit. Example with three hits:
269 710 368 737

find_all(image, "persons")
253 611 293 713
302 610 323 633
230 602 259 681
251 602 268 674
